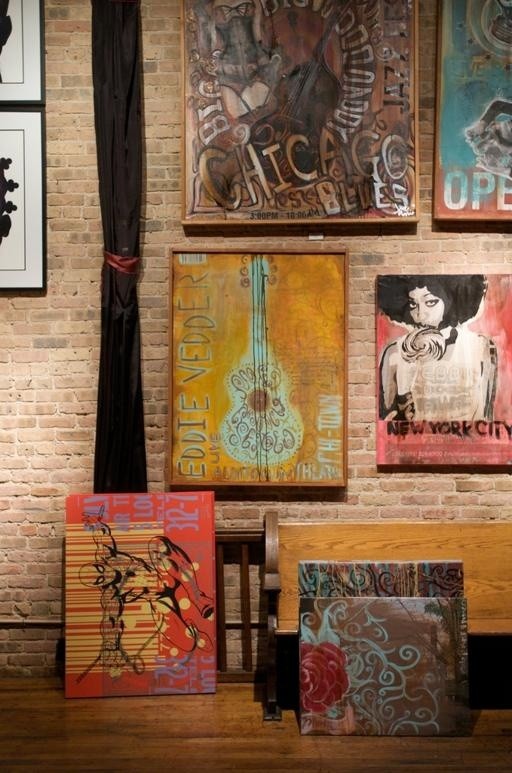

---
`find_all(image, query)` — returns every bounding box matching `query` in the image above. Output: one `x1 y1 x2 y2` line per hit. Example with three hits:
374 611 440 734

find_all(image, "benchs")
260 506 512 722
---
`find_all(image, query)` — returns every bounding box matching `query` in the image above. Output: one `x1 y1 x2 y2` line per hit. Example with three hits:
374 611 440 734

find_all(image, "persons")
376 274 498 424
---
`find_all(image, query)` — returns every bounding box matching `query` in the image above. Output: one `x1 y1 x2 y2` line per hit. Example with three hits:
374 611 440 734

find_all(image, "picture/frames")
165 243 353 490
177 1 426 228
0 105 49 297
430 1 512 223
0 0 47 106
